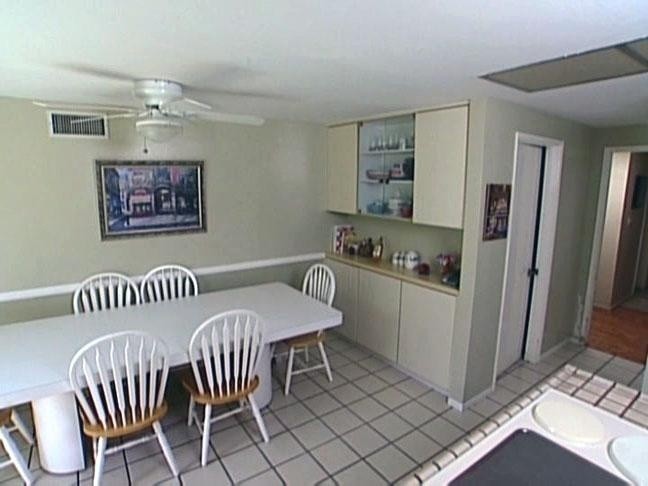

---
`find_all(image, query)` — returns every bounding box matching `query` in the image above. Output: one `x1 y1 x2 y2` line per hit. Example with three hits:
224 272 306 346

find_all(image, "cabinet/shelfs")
402 281 459 393
328 259 401 369
358 113 414 223
415 105 467 229
328 122 358 213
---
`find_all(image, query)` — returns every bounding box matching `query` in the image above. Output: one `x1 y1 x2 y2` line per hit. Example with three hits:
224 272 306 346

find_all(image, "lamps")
134 116 183 152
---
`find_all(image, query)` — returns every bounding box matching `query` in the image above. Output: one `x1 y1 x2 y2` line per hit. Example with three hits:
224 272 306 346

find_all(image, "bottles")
372 236 383 262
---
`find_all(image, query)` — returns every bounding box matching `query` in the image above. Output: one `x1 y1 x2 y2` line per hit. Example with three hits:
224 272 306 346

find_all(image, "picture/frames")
96 160 207 240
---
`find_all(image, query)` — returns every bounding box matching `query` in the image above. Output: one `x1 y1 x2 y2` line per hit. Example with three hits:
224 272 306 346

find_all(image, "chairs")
0 408 35 486
180 309 269 468
270 264 336 396
69 330 185 485
140 266 199 303
73 272 140 314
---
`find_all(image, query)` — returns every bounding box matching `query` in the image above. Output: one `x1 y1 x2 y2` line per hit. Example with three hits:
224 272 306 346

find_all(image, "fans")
31 79 264 126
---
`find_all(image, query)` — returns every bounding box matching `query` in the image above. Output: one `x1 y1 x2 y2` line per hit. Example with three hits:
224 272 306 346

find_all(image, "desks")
0 282 343 474
